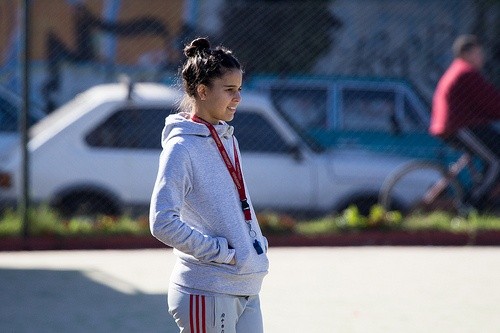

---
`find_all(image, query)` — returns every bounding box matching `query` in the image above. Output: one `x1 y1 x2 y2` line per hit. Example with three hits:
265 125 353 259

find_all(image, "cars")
1 84 446 220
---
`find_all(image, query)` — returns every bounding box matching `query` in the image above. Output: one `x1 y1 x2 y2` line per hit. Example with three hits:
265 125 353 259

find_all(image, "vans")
166 72 485 199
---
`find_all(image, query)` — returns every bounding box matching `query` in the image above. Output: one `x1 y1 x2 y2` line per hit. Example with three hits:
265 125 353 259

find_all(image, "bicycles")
380 138 500 219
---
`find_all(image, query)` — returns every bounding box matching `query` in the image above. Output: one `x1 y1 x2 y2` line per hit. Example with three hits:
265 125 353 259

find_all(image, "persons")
149 37 270 333
430 34 500 219
